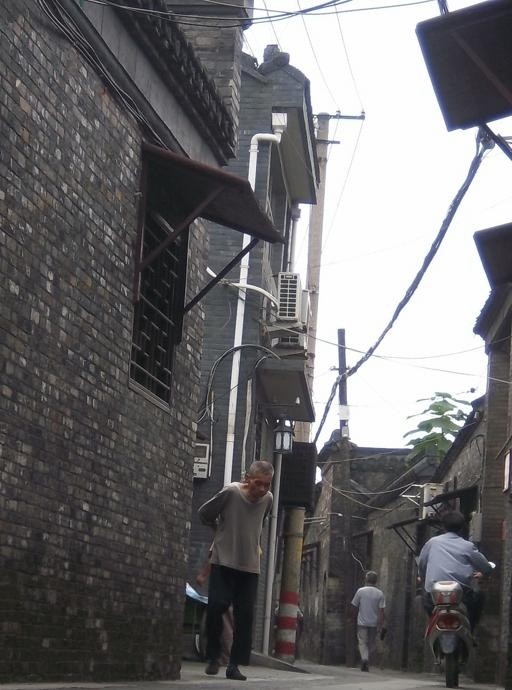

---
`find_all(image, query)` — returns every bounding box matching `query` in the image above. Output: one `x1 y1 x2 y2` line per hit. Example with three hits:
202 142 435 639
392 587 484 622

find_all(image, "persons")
418 511 492 634
351 571 386 671
198 536 262 666
197 459 275 680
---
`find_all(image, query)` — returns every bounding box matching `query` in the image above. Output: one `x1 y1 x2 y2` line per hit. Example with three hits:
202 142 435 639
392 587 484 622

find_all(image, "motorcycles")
423 561 495 688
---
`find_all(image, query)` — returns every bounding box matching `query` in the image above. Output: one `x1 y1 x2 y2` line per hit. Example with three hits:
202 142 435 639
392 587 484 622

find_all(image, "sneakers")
361 661 368 671
206 662 220 675
226 665 246 680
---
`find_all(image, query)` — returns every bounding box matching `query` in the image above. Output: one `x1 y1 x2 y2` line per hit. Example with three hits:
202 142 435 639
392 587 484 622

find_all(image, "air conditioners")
277 273 303 322
